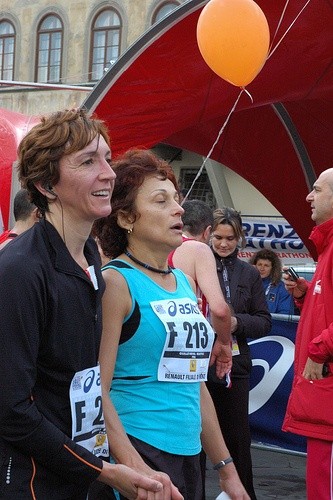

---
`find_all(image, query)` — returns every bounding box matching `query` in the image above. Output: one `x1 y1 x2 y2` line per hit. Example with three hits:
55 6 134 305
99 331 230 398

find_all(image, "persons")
280 167 333 500
248 249 294 315
0 108 272 500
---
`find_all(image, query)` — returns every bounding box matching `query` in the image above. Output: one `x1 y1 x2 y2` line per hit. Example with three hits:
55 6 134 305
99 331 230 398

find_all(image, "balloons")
196 0 270 89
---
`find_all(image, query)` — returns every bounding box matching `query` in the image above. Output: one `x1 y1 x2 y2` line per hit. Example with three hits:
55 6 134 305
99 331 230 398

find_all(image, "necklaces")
124 248 172 275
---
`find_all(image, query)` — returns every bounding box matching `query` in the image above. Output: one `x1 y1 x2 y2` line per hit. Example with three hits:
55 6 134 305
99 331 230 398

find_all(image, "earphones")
45 184 58 197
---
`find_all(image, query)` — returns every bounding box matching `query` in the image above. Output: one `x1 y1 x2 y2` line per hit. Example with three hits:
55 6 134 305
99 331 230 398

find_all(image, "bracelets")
214 456 233 471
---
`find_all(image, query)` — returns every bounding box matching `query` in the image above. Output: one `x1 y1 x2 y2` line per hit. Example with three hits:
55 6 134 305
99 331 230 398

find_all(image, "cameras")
284 267 299 282
